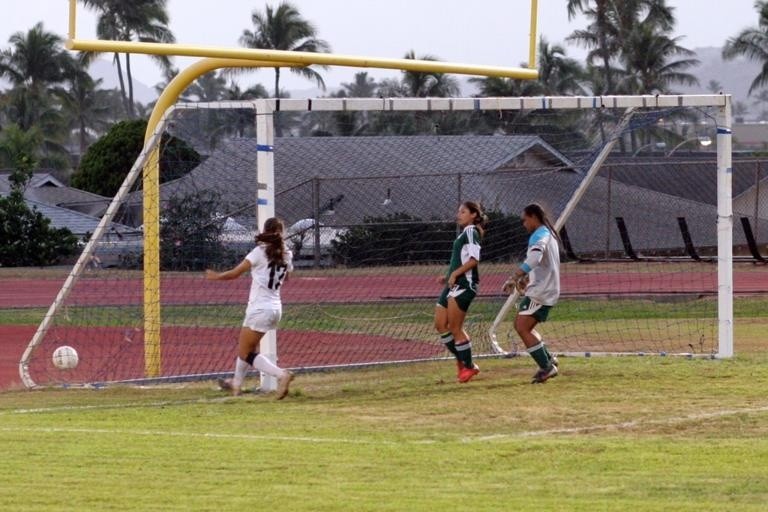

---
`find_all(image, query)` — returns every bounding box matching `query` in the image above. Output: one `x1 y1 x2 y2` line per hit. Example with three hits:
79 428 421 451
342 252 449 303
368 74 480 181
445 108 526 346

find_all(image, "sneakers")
218 376 243 396
534 357 559 378
531 366 558 385
456 361 480 383
272 370 295 401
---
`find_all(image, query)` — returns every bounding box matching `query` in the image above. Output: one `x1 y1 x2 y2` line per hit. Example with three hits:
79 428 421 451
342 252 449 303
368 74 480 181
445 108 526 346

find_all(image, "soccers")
52 346 79 369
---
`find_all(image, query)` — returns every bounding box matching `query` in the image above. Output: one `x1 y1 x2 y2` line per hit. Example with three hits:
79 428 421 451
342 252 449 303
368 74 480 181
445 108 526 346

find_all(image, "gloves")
500 276 517 296
516 278 529 295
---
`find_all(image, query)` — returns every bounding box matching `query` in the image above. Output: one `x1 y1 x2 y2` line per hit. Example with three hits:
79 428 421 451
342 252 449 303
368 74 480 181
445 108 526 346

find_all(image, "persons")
431 200 488 384
203 216 296 404
500 202 564 385
